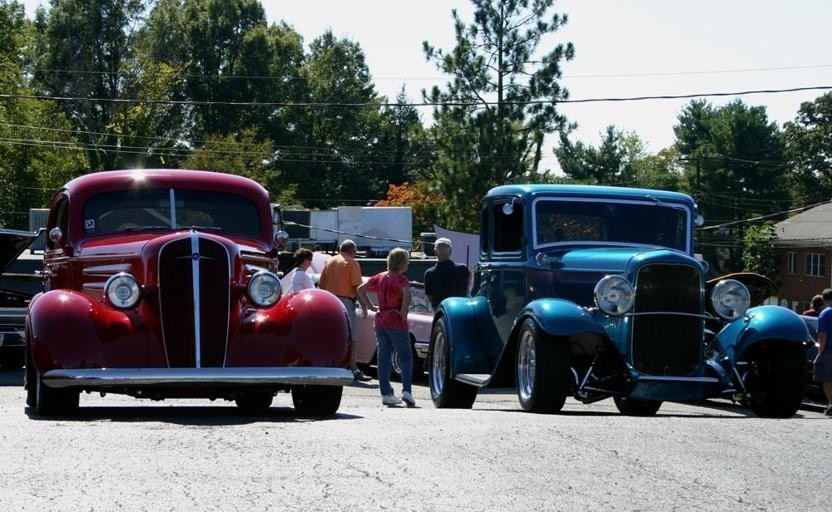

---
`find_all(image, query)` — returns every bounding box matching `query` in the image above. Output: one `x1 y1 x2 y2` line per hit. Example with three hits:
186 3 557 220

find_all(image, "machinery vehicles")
275 205 438 290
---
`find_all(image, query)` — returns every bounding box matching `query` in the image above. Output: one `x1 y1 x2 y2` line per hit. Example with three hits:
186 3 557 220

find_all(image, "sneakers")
382 395 402 404
402 391 415 405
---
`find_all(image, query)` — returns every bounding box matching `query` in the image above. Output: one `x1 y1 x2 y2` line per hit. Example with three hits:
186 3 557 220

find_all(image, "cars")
0 220 57 359
428 184 815 418
282 228 479 377
21 165 351 425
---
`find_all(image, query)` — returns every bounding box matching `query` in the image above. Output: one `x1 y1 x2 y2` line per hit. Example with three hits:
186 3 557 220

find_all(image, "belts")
337 295 354 301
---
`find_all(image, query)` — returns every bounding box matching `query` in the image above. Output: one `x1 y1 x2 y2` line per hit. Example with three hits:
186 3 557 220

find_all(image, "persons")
802 295 824 318
354 246 416 407
289 247 317 294
813 287 831 415
316 238 373 382
425 236 474 317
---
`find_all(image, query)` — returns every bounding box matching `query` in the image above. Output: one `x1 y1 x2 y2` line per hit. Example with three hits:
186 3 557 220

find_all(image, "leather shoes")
355 371 372 381
